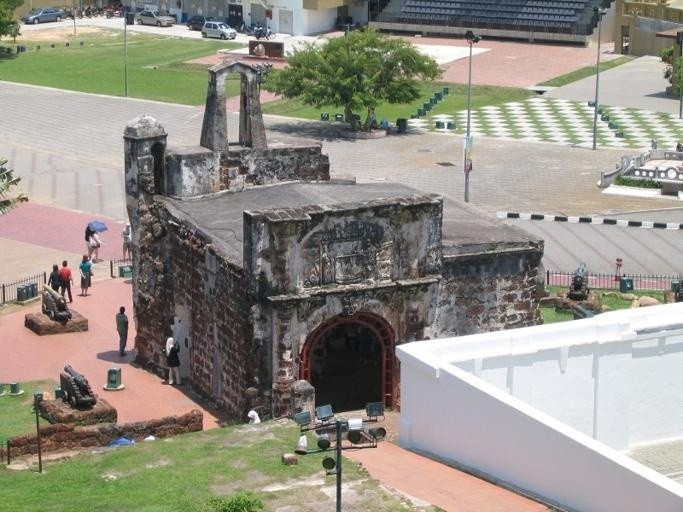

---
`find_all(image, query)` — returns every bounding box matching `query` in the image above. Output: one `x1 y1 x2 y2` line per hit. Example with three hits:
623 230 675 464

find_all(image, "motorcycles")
64 4 122 21
238 24 275 41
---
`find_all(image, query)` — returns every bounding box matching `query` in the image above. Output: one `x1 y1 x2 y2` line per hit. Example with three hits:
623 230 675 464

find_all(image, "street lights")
462 29 480 205
281 400 387 511
31 392 49 473
589 6 607 149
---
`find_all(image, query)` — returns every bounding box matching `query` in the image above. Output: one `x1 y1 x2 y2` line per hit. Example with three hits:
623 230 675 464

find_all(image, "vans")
201 21 236 41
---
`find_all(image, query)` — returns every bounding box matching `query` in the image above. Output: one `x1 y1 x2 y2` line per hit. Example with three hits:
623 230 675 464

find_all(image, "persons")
46 263 63 294
245 409 262 425
85 231 102 264
58 259 75 306
118 224 133 262
114 306 128 358
163 336 186 387
77 254 94 297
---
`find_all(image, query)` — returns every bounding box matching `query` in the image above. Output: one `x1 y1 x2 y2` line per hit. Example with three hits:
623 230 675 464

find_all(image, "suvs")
186 16 215 31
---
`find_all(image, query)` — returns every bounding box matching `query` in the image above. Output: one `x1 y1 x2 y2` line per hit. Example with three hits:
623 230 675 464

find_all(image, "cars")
20 8 68 24
135 9 174 27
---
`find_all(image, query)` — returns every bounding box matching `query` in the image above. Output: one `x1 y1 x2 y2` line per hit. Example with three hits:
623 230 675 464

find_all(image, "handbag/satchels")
89 265 95 276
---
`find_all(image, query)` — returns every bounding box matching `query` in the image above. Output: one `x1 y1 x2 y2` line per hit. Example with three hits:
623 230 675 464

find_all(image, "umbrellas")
83 218 108 239
